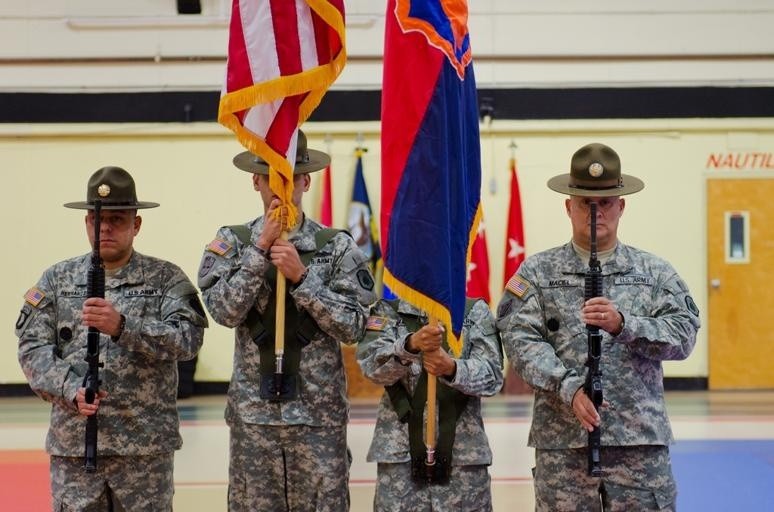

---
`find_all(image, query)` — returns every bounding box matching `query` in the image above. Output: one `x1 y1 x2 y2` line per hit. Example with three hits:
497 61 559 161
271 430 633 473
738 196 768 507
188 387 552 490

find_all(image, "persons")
197 131 378 512
12 165 208 512
351 282 506 512
494 143 702 512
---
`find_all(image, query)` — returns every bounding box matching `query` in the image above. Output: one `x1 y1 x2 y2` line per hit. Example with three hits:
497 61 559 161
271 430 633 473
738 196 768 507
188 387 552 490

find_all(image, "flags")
217 1 348 210
374 3 479 358
318 161 330 228
502 168 525 297
466 203 492 307
345 154 381 282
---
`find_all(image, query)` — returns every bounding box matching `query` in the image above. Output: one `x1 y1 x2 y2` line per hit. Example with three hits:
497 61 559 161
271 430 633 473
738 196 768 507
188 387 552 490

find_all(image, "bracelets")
292 268 309 288
611 312 624 336
117 314 125 334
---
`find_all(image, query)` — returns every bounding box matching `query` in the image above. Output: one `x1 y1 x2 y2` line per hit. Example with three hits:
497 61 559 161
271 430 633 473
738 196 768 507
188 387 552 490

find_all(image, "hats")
546 142 645 198
63 166 161 212
232 127 331 177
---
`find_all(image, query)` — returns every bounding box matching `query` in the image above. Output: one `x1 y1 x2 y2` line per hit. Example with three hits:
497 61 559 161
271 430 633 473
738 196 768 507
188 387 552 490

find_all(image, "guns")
83 200 104 472
585 202 603 477
